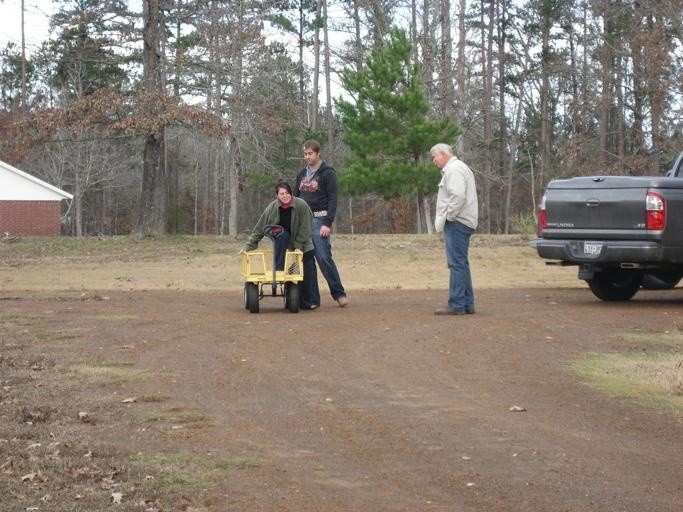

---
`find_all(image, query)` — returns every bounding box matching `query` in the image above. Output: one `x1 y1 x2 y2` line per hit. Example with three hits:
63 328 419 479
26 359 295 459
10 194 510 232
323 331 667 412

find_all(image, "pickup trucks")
536 150 683 300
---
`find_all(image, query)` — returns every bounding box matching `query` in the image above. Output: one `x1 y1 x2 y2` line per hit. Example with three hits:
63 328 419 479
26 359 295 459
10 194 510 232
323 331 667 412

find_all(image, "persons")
430 143 479 315
237 181 317 310
294 139 349 307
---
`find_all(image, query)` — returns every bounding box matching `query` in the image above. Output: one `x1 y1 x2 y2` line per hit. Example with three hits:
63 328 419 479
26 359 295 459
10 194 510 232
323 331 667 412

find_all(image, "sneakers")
298 298 321 311
465 308 475 314
433 306 465 317
336 296 349 307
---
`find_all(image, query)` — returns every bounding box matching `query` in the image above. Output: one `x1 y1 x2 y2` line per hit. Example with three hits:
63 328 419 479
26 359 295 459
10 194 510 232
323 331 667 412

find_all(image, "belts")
312 211 327 218
